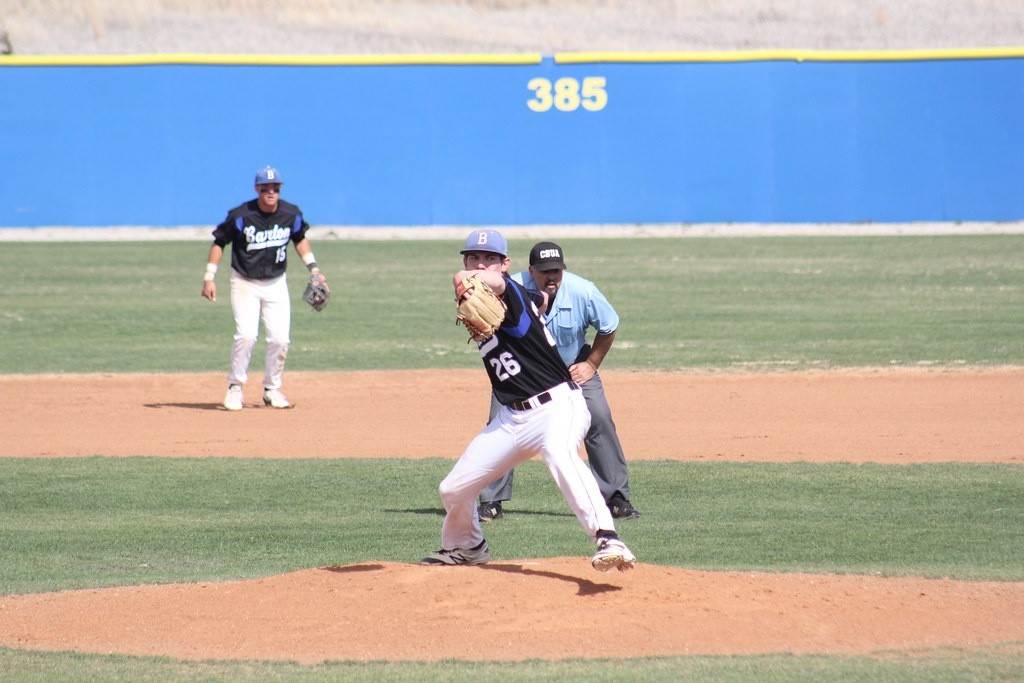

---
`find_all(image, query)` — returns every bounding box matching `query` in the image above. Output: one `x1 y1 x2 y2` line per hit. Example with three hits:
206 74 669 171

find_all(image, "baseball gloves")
302 273 330 313
453 273 509 347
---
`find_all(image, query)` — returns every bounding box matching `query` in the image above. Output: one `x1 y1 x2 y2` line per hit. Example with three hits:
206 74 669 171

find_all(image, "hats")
529 242 567 271
460 229 508 258
255 167 283 186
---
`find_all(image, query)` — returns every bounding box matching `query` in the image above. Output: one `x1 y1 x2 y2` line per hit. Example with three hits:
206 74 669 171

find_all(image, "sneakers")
477 500 503 522
592 537 637 573
224 385 244 410
263 390 289 408
419 538 489 566
607 494 640 520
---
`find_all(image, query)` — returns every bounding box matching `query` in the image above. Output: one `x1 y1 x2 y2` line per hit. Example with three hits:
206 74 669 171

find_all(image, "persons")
201 165 330 411
419 228 640 572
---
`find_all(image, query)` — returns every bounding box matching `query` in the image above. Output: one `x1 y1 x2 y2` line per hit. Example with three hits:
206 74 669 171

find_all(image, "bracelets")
307 262 317 272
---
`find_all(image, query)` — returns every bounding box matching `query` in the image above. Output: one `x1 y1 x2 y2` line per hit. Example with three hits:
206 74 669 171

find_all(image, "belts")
509 381 577 411
